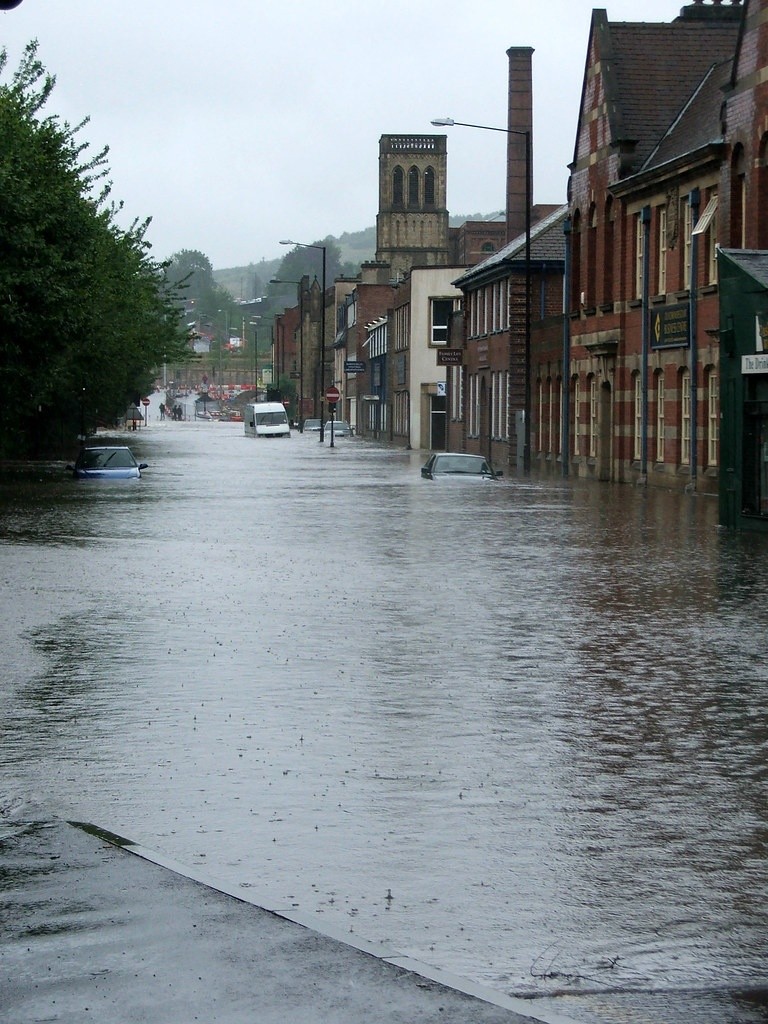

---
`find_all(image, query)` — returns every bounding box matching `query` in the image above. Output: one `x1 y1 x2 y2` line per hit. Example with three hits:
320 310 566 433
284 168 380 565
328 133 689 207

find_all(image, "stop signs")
326 387 339 402
142 399 150 406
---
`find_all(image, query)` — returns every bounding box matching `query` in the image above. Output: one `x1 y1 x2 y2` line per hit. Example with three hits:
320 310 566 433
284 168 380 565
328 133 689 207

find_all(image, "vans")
244 403 291 438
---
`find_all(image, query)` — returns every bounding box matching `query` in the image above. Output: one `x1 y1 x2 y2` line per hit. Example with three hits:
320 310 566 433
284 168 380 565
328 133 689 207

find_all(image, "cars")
304 419 325 431
324 421 353 437
420 453 504 481
65 446 148 479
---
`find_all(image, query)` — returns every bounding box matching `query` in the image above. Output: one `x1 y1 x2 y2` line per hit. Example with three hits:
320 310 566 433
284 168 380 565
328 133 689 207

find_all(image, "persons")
159 403 165 419
173 405 182 421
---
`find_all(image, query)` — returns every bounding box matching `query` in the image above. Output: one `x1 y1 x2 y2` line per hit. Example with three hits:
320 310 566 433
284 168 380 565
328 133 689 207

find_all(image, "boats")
210 411 222 418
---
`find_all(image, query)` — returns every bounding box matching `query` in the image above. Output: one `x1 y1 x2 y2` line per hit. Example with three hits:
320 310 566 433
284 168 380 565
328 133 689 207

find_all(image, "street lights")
280 240 326 442
250 322 273 383
270 280 303 433
230 327 257 403
431 117 532 471
253 316 285 406
164 299 197 387
218 309 227 331
